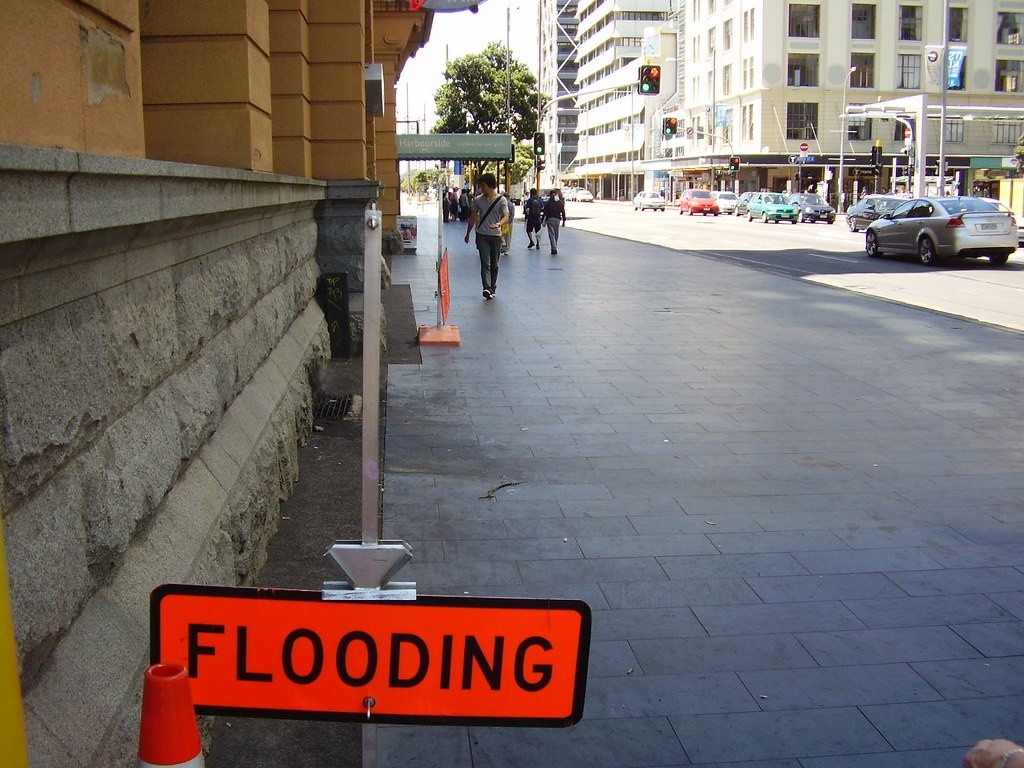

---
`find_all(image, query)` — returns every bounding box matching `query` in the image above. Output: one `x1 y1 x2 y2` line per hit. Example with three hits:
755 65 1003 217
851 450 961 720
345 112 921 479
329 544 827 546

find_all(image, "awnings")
396 134 512 161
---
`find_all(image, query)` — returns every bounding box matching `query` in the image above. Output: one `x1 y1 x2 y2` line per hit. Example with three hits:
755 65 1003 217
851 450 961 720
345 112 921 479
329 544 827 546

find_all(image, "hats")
453 187 460 192
502 193 510 197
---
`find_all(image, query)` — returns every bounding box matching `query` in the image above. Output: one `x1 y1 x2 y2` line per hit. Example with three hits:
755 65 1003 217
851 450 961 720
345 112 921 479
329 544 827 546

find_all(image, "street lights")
837 67 857 212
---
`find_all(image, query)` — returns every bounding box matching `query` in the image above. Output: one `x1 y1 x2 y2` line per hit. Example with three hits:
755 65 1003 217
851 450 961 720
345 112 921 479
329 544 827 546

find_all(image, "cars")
711 192 740 215
980 198 1024 244
679 189 720 216
866 196 1020 266
746 192 799 225
846 196 907 231
735 192 753 216
789 193 836 224
633 191 666 212
522 186 594 207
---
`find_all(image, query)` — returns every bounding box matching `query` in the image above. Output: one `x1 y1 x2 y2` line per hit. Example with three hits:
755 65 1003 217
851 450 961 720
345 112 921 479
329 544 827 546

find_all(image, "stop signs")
799 141 808 152
905 128 913 138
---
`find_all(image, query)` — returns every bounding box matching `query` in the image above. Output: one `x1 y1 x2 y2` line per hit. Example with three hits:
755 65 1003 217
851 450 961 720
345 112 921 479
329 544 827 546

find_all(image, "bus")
498 176 521 206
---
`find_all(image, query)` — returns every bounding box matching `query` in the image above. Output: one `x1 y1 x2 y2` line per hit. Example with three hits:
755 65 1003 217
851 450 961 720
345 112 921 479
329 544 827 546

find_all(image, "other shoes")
491 287 496 296
482 289 493 300
536 244 541 250
504 251 509 256
527 241 535 248
501 245 507 254
551 249 557 254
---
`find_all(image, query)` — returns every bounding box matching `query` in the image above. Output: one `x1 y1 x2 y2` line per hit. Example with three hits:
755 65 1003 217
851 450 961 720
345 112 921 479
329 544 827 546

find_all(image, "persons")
465 173 509 299
542 190 566 254
501 193 515 254
863 187 867 196
525 188 543 248
883 187 905 195
443 187 482 223
522 190 530 205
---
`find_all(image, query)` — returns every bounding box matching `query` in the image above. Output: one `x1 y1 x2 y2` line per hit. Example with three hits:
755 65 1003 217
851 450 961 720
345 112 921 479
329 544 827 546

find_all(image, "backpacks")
530 195 541 217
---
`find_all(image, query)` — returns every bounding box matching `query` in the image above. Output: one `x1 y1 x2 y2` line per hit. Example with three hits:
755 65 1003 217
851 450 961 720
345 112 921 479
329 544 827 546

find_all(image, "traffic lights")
664 118 678 135
729 158 741 174
639 66 661 93
534 132 545 155
536 157 546 173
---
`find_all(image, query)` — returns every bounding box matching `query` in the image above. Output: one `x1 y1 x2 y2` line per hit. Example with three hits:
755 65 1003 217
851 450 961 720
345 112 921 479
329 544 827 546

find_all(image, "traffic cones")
138 662 206 767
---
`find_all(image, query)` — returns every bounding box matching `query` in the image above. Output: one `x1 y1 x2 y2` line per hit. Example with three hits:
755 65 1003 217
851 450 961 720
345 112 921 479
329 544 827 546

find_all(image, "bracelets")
496 223 501 228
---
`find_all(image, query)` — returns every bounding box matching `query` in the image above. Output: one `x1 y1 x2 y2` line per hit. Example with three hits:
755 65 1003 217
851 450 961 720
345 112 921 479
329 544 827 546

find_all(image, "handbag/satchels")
500 223 510 235
